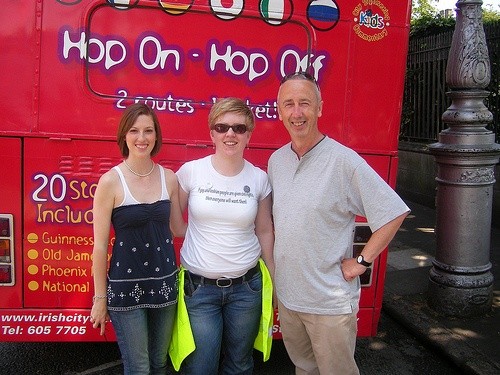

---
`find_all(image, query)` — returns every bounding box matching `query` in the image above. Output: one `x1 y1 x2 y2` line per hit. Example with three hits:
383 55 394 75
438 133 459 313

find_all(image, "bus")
0 0 412 344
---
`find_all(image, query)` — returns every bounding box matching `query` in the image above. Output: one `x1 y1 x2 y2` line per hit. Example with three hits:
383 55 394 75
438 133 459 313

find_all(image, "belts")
183 263 261 288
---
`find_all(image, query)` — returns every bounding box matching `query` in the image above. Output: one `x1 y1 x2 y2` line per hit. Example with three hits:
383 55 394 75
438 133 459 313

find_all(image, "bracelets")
95 296 107 298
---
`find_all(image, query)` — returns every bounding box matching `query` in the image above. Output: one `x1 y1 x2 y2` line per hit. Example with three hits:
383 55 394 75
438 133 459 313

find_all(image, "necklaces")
123 160 155 176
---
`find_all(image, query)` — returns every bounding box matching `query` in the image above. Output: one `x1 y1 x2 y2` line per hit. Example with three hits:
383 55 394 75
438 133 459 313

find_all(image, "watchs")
357 255 372 267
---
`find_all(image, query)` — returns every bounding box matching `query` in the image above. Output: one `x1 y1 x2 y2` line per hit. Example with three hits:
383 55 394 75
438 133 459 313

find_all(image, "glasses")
210 123 250 134
279 71 319 90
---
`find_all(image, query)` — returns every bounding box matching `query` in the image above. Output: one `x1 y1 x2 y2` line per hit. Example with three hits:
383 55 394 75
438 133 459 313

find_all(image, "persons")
176 97 273 375
90 103 189 375
268 73 410 375
